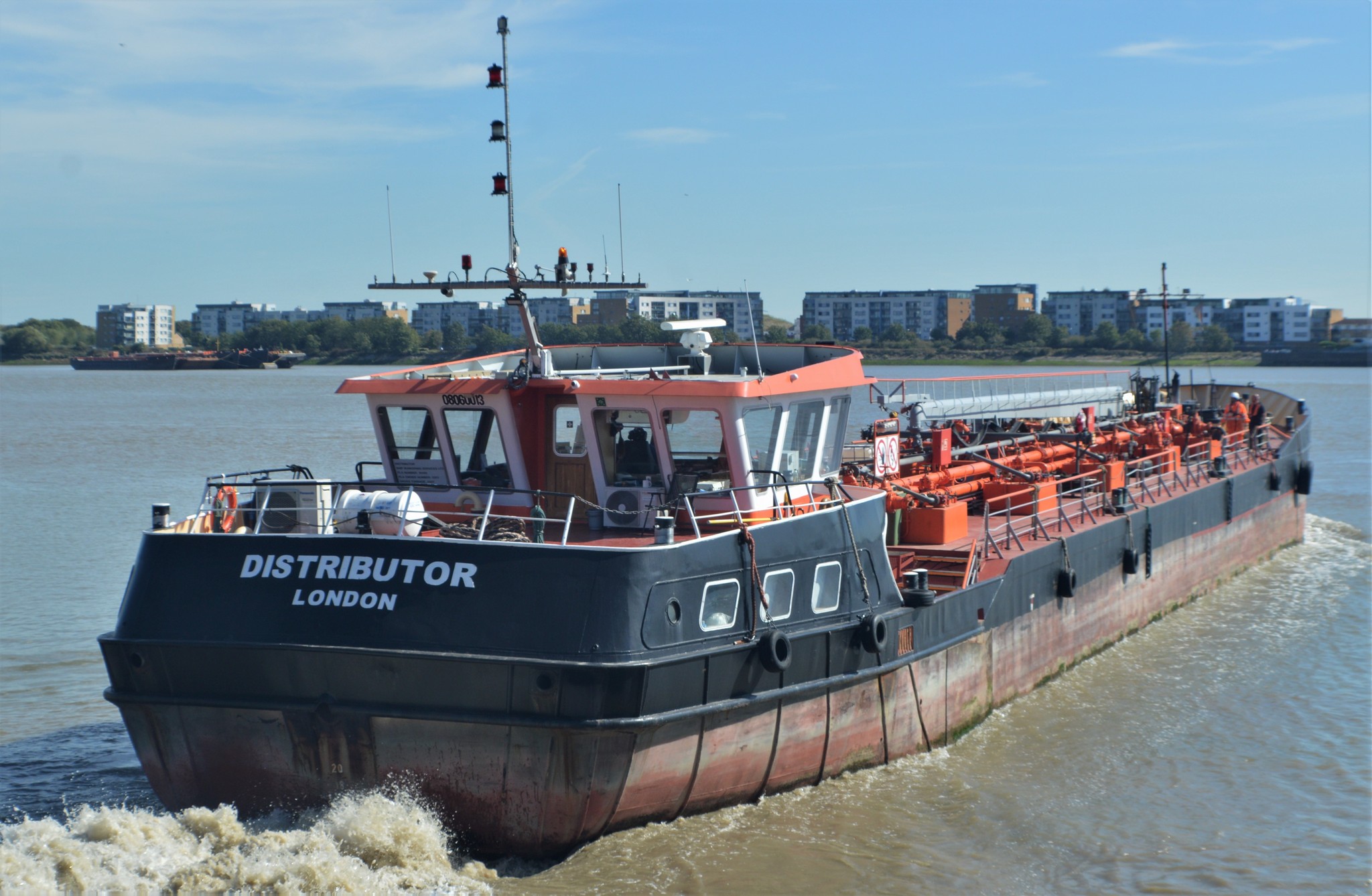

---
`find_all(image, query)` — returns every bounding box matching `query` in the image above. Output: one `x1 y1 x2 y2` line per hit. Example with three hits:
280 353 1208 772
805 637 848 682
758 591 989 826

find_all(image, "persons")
1221 392 1250 453
1249 394 1265 450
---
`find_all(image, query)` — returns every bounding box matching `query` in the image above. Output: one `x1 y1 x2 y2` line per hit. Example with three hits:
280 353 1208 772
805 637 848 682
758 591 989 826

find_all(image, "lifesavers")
1061 568 1077 597
1300 461 1313 494
760 630 792 673
861 615 888 653
901 589 934 606
1126 548 1139 574
211 486 237 532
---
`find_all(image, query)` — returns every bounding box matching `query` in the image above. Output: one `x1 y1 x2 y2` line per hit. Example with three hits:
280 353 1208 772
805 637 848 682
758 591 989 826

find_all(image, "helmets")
1230 392 1241 400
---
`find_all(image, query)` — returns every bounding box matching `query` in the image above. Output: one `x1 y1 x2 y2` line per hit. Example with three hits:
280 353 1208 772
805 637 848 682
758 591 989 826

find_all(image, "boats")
97 14 1316 861
69 346 306 372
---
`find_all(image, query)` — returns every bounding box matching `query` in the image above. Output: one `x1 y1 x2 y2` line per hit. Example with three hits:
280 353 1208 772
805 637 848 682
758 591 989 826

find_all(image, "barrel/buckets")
585 509 605 530
333 489 428 536
